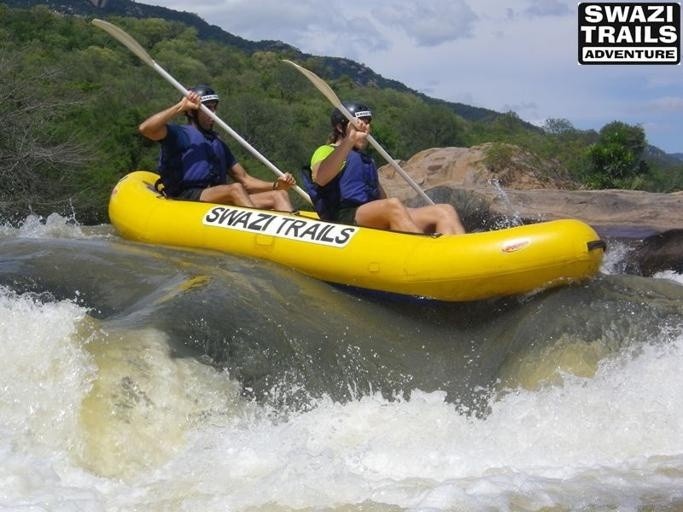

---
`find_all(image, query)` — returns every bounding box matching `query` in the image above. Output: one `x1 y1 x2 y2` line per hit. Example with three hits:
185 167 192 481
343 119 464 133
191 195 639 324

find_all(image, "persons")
137 86 297 214
309 102 468 236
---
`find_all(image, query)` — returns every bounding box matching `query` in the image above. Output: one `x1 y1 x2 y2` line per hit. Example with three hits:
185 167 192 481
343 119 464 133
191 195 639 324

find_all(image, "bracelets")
272 181 279 189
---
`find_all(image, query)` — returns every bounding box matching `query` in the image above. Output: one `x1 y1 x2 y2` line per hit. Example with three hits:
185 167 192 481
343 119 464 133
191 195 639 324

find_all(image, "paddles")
281 59 434 207
91 19 313 206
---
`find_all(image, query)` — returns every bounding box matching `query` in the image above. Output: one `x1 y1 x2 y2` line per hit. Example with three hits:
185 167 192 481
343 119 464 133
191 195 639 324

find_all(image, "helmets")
331 101 372 128
180 85 220 117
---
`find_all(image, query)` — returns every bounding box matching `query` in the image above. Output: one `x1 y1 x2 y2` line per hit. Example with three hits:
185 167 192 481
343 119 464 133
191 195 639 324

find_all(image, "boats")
107 171 609 308
